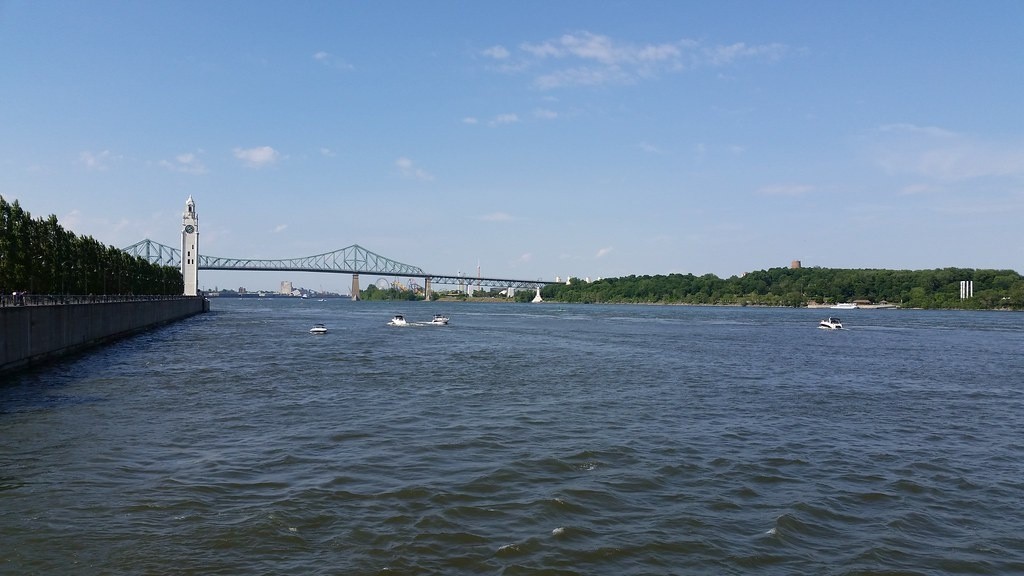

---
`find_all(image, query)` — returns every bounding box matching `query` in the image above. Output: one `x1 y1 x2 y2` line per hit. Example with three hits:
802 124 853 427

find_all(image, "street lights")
118 270 129 295
156 278 164 296
31 256 45 295
149 276 157 295
103 268 114 297
131 271 141 295
164 279 184 295
141 274 149 294
85 265 97 297
62 260 74 297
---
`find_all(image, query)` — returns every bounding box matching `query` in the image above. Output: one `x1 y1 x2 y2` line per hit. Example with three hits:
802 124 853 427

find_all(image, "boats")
431 315 451 325
318 299 326 301
391 315 406 324
309 323 327 333
831 303 859 309
820 317 843 329
301 294 310 299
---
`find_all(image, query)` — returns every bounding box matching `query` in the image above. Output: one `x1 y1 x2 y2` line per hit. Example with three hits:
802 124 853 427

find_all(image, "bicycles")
12 291 27 306
